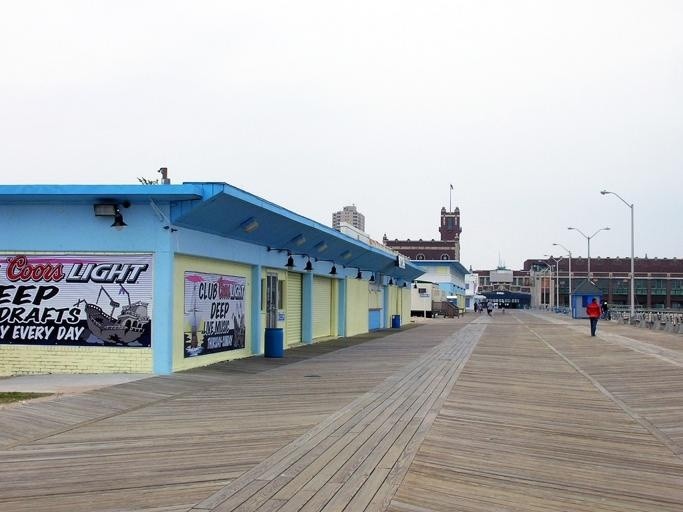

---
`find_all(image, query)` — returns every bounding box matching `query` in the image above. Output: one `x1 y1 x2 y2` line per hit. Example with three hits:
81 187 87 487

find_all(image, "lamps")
267 234 417 288
240 217 258 234
110 206 128 231
94 204 118 216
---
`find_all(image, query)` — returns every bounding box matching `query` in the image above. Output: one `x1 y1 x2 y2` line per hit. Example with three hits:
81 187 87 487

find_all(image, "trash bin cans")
265 328 283 358
392 315 400 328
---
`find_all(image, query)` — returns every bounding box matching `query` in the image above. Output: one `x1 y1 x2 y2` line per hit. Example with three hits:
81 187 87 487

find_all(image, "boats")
73 284 148 344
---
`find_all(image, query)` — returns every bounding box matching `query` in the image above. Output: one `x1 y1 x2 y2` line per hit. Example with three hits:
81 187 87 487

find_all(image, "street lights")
553 244 571 311
601 190 635 317
533 260 554 307
544 255 562 309
568 227 610 274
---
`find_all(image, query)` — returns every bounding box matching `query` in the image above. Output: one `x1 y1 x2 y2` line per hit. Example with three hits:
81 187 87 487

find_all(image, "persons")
473 300 505 316
585 297 603 336
600 301 608 319
